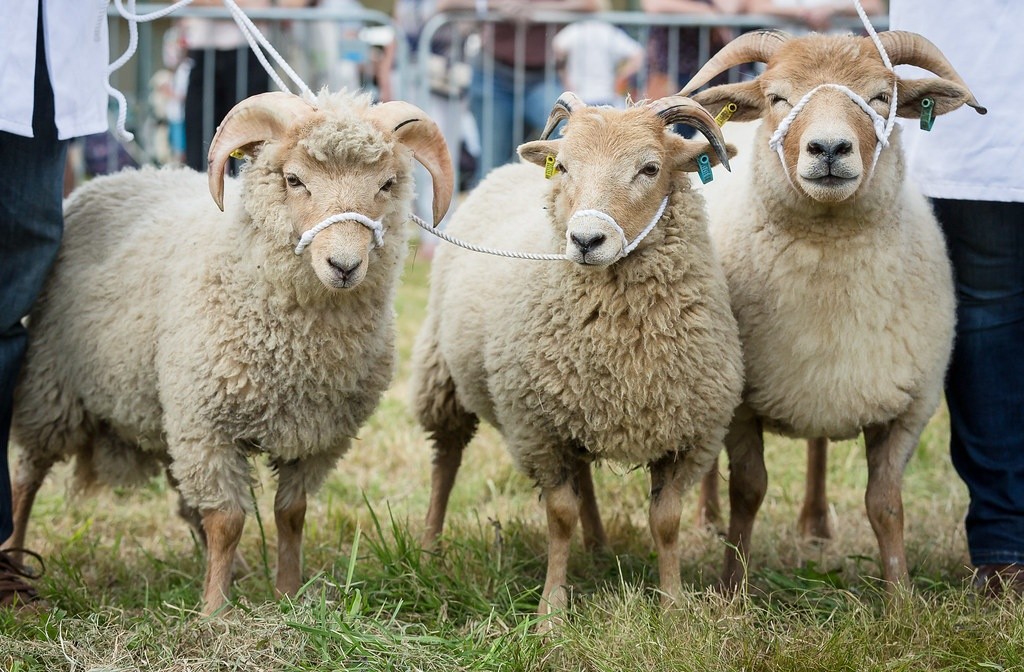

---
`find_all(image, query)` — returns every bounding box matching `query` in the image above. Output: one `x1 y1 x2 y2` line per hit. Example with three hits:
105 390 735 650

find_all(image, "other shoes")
0 547 47 612
964 562 1024 605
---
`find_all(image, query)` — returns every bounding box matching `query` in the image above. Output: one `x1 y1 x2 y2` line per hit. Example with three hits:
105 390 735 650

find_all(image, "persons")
0 0 108 622
140 0 888 265
888 0 1024 600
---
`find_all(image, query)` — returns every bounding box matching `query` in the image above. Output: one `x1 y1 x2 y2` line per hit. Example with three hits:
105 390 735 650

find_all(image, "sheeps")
0 80 455 631
407 89 749 650
668 24 990 639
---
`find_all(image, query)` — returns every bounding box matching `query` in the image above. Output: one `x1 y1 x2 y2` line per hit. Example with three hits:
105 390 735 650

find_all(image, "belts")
493 56 559 73
